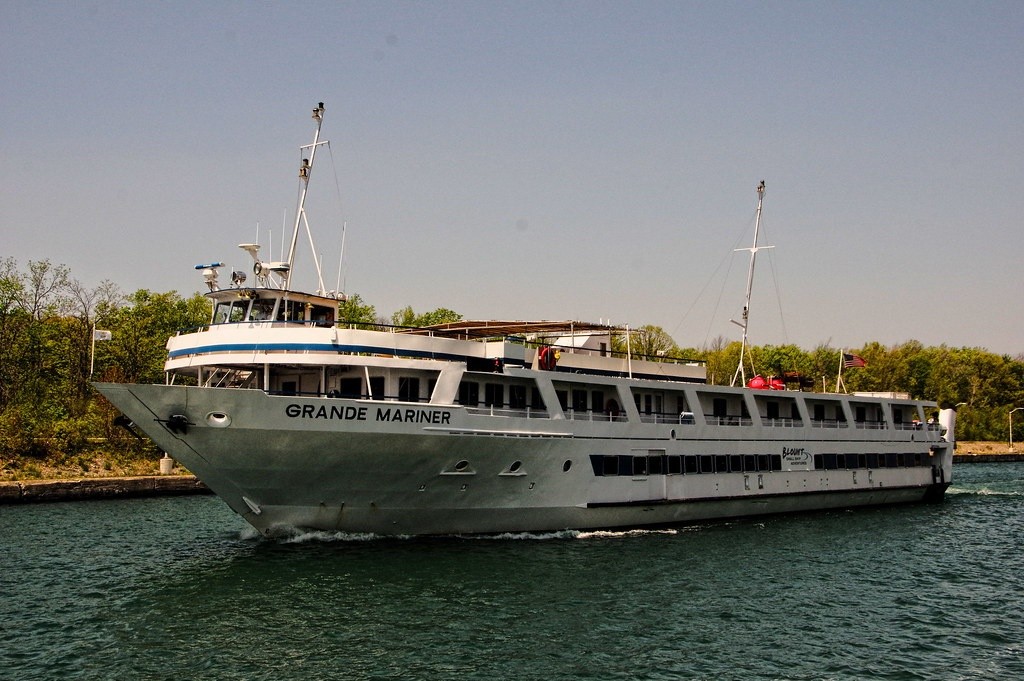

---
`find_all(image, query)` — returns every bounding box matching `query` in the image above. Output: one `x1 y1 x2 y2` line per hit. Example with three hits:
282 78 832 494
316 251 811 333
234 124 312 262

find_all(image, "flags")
843 354 865 369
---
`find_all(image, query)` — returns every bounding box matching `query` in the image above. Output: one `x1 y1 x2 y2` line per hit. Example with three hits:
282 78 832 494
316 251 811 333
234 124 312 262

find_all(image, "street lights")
1009 408 1023 454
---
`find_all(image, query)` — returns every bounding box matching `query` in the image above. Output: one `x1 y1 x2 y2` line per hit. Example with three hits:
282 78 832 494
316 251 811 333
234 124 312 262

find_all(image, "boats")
83 98 962 546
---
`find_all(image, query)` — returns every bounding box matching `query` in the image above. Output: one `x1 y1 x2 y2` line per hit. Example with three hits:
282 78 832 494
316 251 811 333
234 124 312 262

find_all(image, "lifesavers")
541 347 556 371
